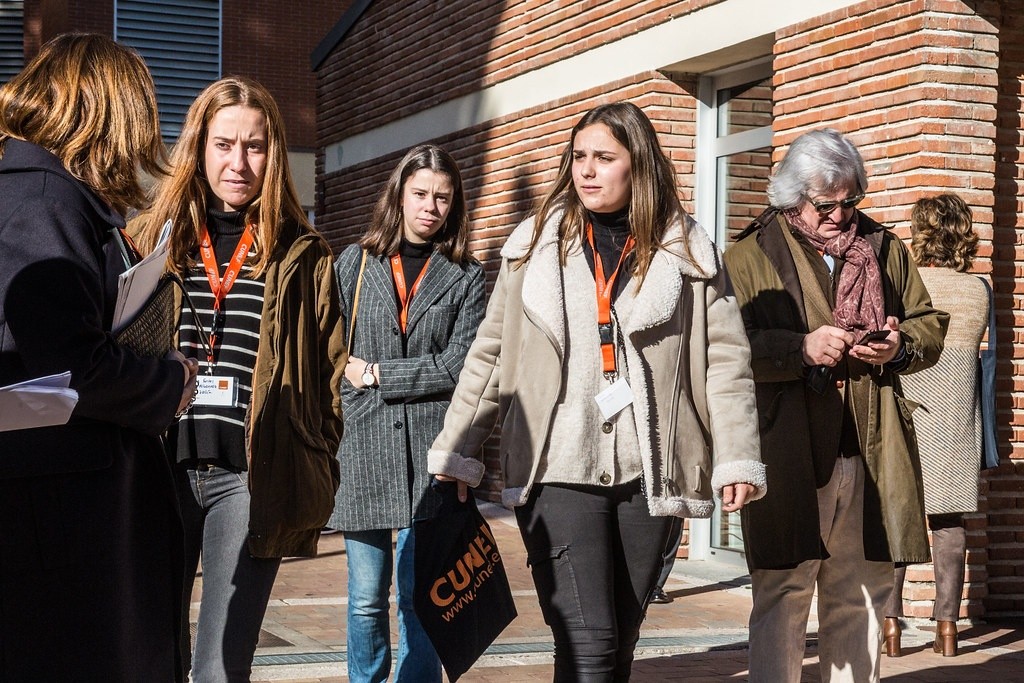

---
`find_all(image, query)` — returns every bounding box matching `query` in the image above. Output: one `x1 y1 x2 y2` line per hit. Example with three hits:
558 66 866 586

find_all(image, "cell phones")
855 330 891 346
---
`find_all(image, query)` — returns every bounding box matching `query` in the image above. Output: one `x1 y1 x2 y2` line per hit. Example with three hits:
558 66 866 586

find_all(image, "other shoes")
650 589 674 603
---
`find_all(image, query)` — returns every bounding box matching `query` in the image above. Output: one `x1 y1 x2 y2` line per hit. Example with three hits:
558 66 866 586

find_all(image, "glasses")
802 192 866 215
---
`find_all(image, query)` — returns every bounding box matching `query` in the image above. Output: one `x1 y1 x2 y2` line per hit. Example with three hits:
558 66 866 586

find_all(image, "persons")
0 31 191 682
429 103 766 683
118 79 350 683
722 128 950 683
878 191 994 658
650 516 685 604
334 142 487 683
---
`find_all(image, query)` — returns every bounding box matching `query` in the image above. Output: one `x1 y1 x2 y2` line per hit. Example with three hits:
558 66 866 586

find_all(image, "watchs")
361 363 375 389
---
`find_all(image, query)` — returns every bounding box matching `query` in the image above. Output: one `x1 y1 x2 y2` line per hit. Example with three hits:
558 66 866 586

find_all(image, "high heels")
933 621 960 657
881 616 902 657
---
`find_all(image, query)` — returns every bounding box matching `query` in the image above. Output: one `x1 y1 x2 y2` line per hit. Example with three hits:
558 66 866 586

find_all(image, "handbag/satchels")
975 275 1000 471
412 480 518 683
109 271 215 360
